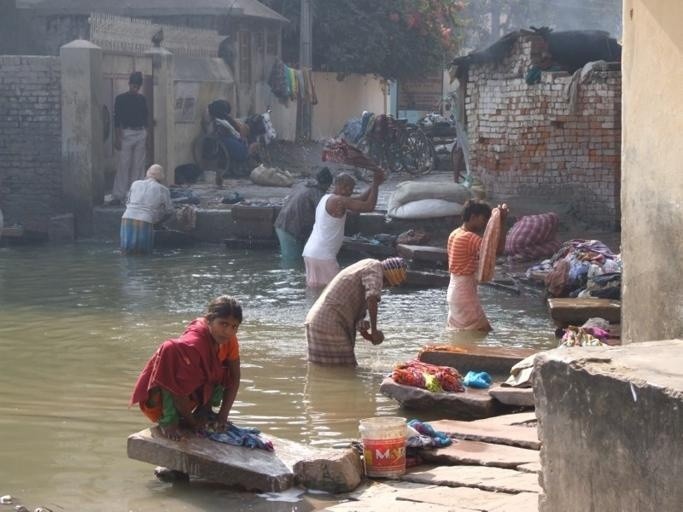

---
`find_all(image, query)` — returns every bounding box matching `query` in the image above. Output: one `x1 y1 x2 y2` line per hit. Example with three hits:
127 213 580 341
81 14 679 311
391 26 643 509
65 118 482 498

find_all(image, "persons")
274 167 333 264
140 294 242 441
110 72 151 205
304 257 407 367
445 198 508 331
301 169 385 287
120 164 176 255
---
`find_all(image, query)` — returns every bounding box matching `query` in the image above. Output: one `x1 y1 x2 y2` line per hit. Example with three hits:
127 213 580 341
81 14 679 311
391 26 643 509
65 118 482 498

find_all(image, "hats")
381 257 406 286
146 163 164 181
316 167 332 184
129 73 143 85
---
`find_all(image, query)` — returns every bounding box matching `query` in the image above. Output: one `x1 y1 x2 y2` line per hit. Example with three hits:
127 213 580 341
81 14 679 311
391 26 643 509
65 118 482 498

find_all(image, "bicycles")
355 115 436 182
192 120 273 178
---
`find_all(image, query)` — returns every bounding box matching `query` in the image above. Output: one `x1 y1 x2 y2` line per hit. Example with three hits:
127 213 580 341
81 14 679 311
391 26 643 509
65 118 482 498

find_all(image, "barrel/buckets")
204 170 217 183
356 418 409 477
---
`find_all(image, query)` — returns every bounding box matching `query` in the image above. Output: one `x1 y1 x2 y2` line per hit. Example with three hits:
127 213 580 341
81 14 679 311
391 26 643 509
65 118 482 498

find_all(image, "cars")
396 110 463 168
